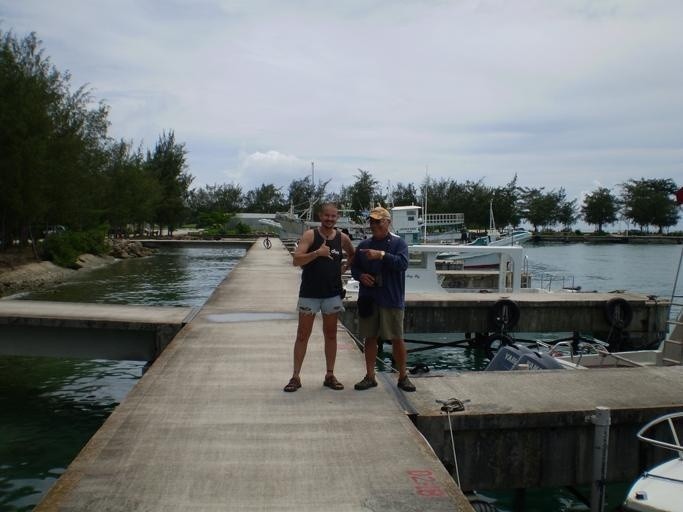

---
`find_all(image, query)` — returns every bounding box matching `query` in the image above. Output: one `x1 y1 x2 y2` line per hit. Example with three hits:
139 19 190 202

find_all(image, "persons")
460 225 469 244
350 207 417 390
283 203 355 391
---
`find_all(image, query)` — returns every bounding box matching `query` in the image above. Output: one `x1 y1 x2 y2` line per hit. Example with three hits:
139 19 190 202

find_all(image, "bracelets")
380 251 385 260
314 251 318 257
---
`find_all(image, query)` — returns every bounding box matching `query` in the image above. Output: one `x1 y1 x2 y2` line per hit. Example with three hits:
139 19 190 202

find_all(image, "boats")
262 156 535 268
615 412 683 512
480 331 664 372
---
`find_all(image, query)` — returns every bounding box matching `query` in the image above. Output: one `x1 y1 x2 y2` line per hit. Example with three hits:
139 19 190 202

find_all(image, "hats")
365 207 391 222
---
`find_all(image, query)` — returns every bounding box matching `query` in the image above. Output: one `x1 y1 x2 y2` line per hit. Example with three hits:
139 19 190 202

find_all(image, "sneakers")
322 374 343 390
283 377 301 392
397 375 416 392
353 374 377 390
